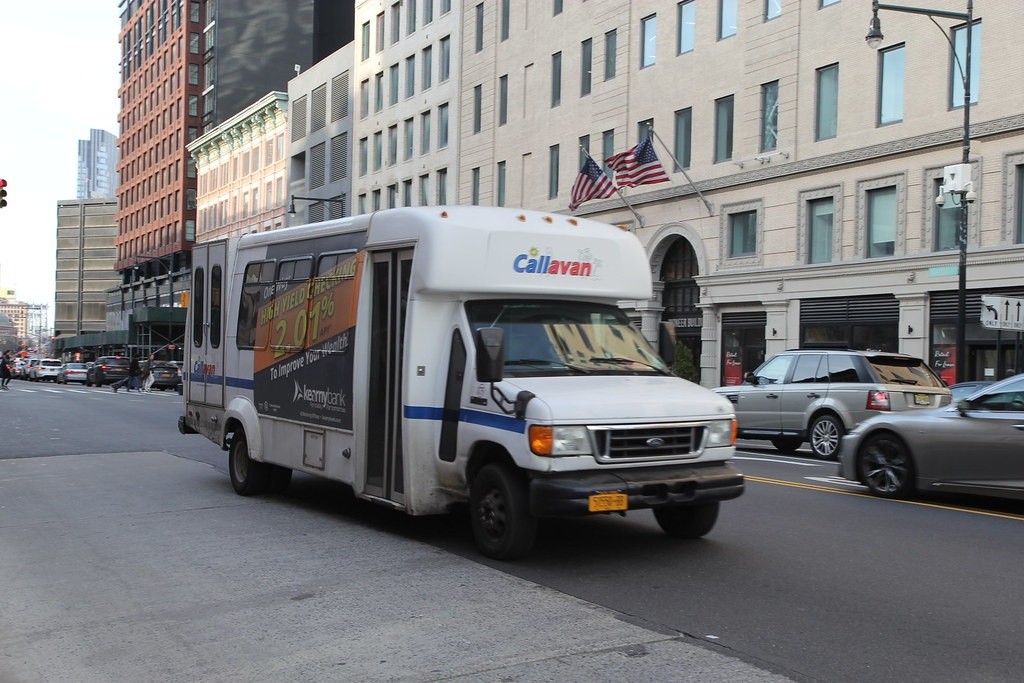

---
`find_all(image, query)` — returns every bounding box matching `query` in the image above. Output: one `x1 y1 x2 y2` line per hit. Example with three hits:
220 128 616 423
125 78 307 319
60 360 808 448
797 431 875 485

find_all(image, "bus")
177 205 745 561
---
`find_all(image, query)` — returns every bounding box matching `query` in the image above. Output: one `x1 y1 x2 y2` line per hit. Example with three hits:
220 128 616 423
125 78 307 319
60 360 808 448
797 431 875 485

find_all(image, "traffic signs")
980 294 1024 333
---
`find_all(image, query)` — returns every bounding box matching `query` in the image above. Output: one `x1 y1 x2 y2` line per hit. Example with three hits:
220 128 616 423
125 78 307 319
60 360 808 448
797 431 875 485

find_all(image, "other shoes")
0 385 8 391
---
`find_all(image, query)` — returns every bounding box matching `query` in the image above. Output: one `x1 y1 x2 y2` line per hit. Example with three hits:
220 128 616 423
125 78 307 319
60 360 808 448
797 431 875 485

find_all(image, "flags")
568 154 618 212
602 134 672 190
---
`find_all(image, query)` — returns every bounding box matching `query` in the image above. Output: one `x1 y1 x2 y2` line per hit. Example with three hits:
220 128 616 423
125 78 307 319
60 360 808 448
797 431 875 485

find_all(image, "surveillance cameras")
935 196 946 207
966 192 977 203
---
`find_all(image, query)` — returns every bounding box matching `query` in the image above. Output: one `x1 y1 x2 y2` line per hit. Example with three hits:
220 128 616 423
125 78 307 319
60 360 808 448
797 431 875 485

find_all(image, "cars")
706 348 951 460
837 374 1022 500
0 346 183 391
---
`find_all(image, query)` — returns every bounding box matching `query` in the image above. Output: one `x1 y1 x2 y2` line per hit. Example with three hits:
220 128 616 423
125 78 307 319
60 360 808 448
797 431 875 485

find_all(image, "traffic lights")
0 178 7 208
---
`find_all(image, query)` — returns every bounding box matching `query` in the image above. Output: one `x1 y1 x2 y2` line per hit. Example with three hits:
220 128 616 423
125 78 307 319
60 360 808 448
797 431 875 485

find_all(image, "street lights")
287 193 343 219
865 1 972 382
132 251 174 340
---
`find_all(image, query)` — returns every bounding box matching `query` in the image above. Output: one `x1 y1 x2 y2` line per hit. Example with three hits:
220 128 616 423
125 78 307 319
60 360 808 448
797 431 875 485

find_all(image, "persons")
126 353 143 393
0 350 15 389
143 354 157 392
